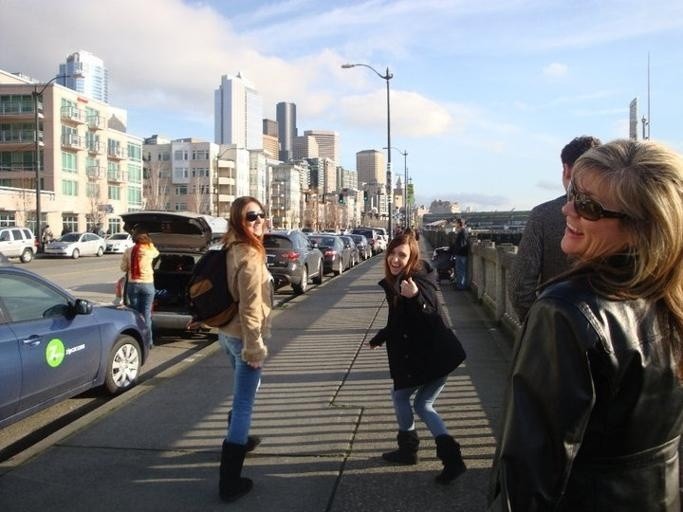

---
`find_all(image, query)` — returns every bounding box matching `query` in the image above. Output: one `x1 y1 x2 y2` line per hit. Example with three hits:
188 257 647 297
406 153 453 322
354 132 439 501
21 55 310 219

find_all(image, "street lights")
216 147 245 216
382 145 408 233
341 62 393 248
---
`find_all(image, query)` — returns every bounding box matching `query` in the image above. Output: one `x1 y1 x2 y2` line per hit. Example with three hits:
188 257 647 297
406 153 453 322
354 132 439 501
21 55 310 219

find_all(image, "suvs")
262 227 324 294
0 226 39 262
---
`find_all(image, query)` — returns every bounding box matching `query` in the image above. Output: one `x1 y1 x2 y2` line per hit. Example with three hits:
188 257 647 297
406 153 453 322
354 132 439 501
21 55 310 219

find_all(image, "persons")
453 218 468 291
507 136 602 326
43 226 52 243
214 194 272 503
363 232 469 484
486 138 683 511
119 227 161 350
393 224 420 244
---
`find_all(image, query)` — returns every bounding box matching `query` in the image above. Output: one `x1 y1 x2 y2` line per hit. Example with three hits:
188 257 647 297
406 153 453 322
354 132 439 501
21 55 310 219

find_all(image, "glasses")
566 180 639 221
245 210 265 223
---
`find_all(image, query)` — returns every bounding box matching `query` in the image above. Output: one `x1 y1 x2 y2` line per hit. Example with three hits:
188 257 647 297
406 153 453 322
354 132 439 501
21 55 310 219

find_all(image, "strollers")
431 246 454 285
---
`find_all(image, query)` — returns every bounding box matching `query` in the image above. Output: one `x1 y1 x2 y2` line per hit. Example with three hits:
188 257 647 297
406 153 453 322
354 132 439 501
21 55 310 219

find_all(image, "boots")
219 439 253 505
381 430 421 466
434 433 467 483
227 411 261 452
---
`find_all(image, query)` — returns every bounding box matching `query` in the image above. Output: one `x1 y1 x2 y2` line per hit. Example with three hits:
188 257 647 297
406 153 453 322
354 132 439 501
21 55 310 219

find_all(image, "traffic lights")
363 191 367 201
338 193 342 203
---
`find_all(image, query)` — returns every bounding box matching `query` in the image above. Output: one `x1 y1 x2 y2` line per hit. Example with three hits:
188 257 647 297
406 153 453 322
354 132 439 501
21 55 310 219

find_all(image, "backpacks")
184 247 238 329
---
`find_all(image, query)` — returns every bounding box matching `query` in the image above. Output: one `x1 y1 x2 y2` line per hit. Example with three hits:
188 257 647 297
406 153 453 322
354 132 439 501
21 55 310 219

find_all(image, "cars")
301 223 388 275
32 72 89 255
44 232 106 259
112 210 274 336
104 232 135 253
0 266 149 430
